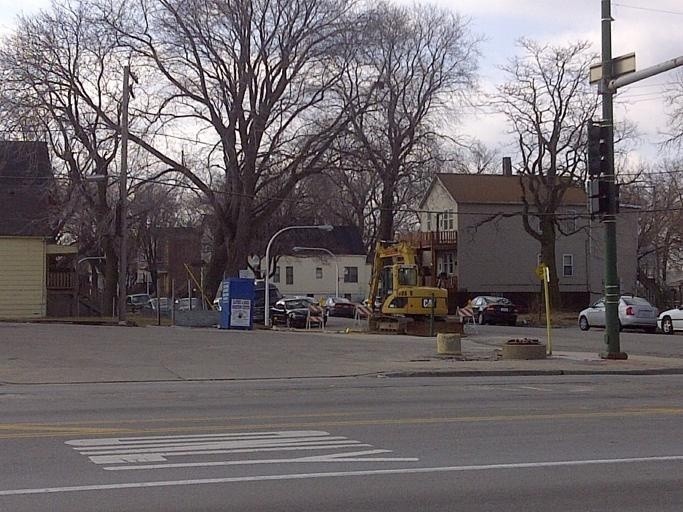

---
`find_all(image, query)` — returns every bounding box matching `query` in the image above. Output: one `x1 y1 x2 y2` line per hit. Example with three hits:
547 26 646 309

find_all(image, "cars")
270 295 397 328
656 304 683 334
578 294 659 332
465 295 518 326
125 293 201 313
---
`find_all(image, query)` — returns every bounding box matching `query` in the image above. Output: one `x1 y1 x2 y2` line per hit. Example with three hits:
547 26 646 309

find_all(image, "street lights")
292 246 339 298
264 224 334 327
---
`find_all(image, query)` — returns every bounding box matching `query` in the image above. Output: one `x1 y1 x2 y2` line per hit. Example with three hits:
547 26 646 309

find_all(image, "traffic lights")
587 123 609 176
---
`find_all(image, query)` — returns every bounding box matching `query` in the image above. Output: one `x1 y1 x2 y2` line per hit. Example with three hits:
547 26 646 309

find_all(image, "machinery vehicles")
367 240 468 338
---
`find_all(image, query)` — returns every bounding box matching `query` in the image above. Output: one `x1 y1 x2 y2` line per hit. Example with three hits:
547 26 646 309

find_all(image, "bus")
214 280 279 323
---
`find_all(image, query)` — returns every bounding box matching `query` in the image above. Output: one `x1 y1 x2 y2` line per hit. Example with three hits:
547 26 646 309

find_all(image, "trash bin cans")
221 278 256 329
